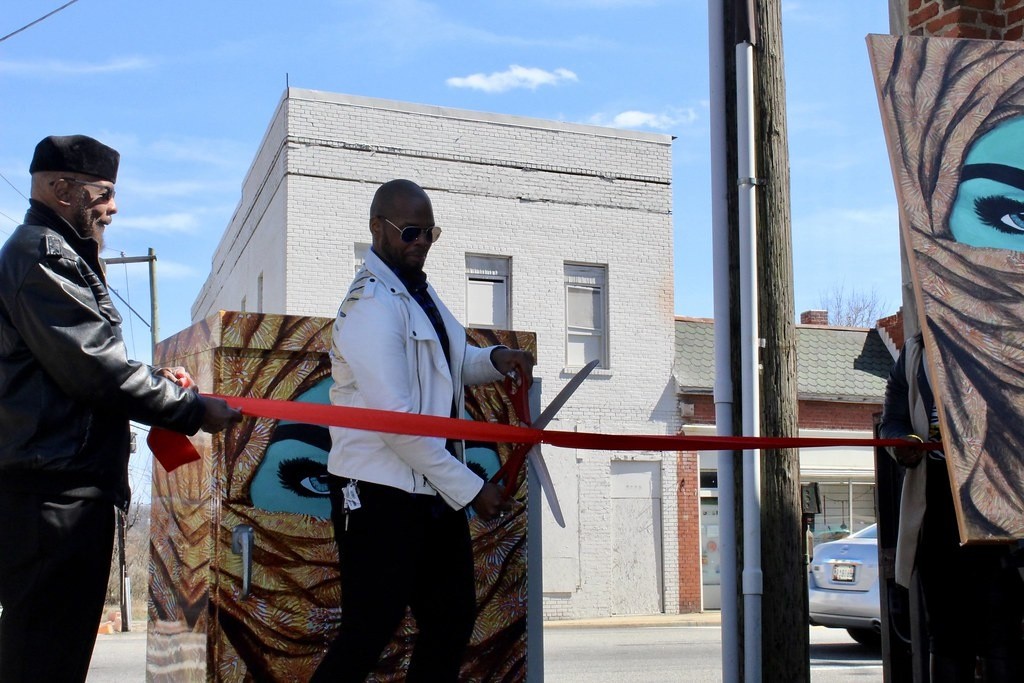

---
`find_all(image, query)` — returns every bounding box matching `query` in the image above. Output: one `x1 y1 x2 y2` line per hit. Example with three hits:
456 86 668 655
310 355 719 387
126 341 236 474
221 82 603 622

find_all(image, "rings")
500 510 508 518
506 370 517 381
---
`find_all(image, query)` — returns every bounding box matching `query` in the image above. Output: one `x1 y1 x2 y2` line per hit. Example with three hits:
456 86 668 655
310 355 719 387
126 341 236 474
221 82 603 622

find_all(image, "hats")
29 135 120 184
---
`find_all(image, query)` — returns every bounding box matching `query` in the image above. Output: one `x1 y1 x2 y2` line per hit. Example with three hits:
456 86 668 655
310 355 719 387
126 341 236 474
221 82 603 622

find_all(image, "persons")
881 328 1024 683
0 135 243 683
309 180 536 683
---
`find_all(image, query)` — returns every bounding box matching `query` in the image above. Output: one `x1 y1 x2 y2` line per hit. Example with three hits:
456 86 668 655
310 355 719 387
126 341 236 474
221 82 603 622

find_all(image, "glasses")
49 177 116 204
377 216 442 243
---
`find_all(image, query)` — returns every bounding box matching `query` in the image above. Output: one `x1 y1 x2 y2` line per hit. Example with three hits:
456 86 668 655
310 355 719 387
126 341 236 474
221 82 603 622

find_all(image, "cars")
808 523 881 648
